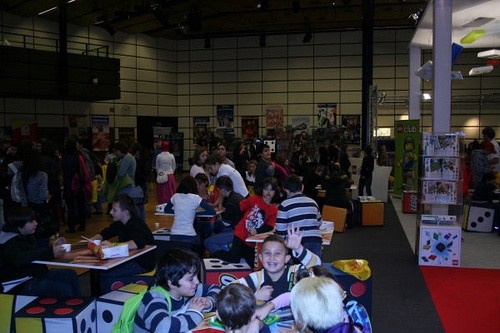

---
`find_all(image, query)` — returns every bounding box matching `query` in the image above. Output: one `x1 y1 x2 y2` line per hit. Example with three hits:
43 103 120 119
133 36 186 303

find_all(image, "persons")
229 177 278 268
89 193 156 295
163 176 217 245
276 175 323 262
130 249 223 333
203 175 247 254
227 222 321 306
214 282 271 333
1 123 380 234
288 275 372 333
0 206 84 301
459 124 500 231
249 264 338 321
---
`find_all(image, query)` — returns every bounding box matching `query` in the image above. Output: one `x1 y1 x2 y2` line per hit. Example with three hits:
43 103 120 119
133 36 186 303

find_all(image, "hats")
116 142 128 155
160 140 170 151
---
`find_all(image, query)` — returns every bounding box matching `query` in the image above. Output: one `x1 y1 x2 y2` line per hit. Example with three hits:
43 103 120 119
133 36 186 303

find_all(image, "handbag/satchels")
155 169 168 183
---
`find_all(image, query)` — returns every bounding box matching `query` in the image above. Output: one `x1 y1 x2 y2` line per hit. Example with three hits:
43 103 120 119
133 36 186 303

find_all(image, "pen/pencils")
81 235 93 243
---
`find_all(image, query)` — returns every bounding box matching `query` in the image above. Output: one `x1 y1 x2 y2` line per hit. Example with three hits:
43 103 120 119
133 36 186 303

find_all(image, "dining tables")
153 204 227 230
32 238 157 295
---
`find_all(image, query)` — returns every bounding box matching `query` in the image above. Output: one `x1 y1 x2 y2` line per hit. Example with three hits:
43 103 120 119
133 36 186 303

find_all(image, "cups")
62 244 71 253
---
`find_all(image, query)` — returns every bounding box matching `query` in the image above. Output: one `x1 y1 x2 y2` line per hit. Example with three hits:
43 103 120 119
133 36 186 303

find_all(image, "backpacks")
70 155 92 201
111 287 172 333
106 161 117 184
